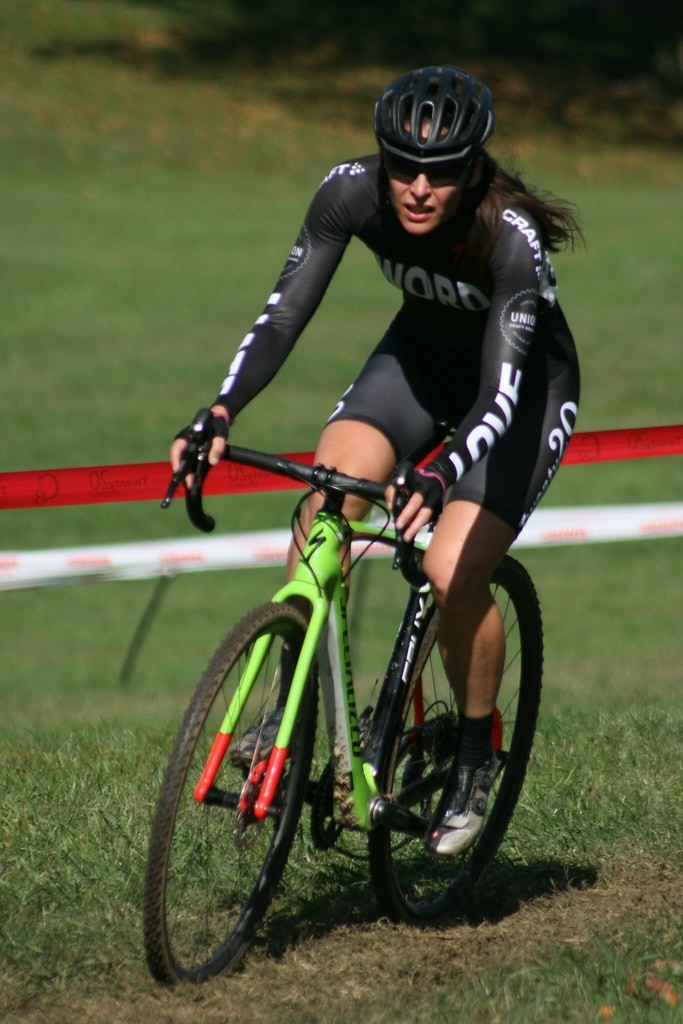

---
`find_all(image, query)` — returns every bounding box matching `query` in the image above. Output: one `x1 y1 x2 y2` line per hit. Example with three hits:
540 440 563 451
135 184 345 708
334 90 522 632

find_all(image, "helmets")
373 63 496 166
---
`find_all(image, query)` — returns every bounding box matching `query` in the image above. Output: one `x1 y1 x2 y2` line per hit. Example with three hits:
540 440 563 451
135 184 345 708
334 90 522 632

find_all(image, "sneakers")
231 702 307 767
425 749 513 861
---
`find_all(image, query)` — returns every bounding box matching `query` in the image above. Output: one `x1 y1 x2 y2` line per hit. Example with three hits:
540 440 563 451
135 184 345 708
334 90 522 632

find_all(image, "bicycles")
141 409 543 989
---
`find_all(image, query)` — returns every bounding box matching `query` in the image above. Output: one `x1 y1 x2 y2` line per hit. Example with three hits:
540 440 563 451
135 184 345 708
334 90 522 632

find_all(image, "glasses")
381 145 478 187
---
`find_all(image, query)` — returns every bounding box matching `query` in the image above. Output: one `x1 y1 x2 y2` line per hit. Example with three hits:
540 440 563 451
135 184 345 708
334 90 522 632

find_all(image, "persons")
171 65 581 858
